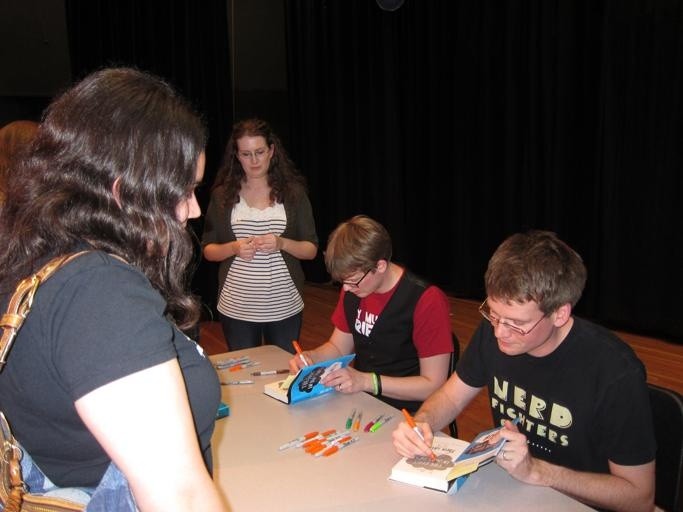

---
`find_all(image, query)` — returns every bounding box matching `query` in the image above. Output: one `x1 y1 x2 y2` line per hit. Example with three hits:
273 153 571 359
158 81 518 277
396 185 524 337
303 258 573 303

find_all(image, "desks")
204 343 598 511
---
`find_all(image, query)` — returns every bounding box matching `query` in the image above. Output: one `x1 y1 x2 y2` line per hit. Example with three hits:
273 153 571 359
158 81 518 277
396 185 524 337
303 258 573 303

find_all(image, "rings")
339 384 343 392
500 450 508 462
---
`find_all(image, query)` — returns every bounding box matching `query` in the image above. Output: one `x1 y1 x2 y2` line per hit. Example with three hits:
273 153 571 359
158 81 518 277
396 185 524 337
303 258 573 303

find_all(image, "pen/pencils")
292 340 309 367
250 368 290 376
278 428 359 457
220 380 254 385
401 407 438 462
364 415 382 432
354 410 363 431
369 416 393 432
346 408 356 428
215 357 260 371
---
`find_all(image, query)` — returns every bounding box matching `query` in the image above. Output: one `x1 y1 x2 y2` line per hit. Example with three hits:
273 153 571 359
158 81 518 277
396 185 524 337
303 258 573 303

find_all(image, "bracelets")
375 372 382 399
372 372 380 398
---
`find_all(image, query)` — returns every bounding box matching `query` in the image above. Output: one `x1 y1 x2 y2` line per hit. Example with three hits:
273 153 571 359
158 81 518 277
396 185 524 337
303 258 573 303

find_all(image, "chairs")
646 383 683 510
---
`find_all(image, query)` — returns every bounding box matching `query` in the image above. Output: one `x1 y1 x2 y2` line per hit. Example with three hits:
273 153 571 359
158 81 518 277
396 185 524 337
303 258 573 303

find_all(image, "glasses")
341 268 370 288
237 151 267 158
479 298 549 336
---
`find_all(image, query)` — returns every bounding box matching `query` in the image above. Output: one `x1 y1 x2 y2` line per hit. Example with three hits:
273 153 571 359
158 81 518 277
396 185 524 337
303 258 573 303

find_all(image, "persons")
287 214 455 419
2 69 227 512
200 118 319 356
0 120 39 206
393 231 658 512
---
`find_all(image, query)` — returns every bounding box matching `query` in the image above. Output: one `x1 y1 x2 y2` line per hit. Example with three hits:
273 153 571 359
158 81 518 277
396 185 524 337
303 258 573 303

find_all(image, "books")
263 354 357 405
389 417 521 498
